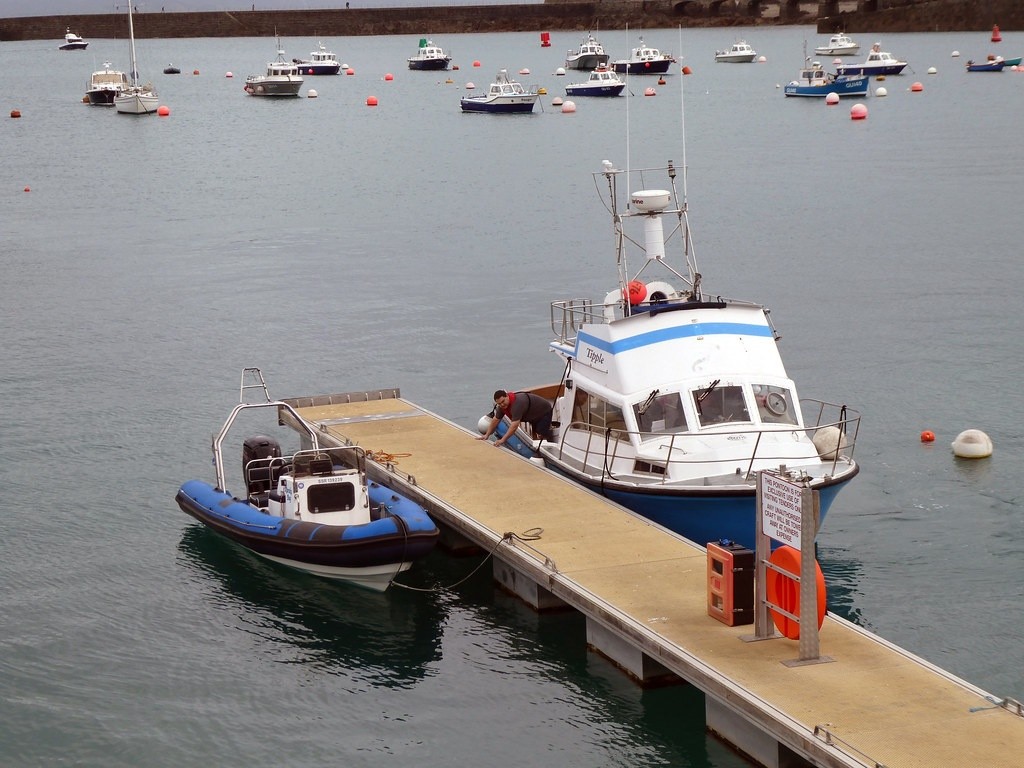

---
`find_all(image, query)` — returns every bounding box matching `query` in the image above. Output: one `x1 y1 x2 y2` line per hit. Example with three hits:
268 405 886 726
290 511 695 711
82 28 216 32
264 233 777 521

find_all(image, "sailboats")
115 0 159 115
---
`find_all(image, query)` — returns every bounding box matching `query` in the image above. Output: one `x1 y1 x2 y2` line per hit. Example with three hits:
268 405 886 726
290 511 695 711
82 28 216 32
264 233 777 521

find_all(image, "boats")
564 62 625 97
783 31 869 97
989 56 1022 66
176 364 444 593
814 32 861 56
86 57 128 106
59 25 90 50
474 26 861 548
407 38 452 72
565 29 610 70
460 69 537 114
291 41 340 76
835 42 908 75
966 60 1005 72
611 36 677 74
713 40 756 63
267 25 299 76
244 74 304 96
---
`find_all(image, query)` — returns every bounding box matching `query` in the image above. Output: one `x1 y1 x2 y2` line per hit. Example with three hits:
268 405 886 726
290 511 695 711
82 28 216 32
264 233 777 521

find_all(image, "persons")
134 6 137 12
346 2 349 9
161 6 166 13
117 6 118 13
474 389 552 446
252 4 255 10
